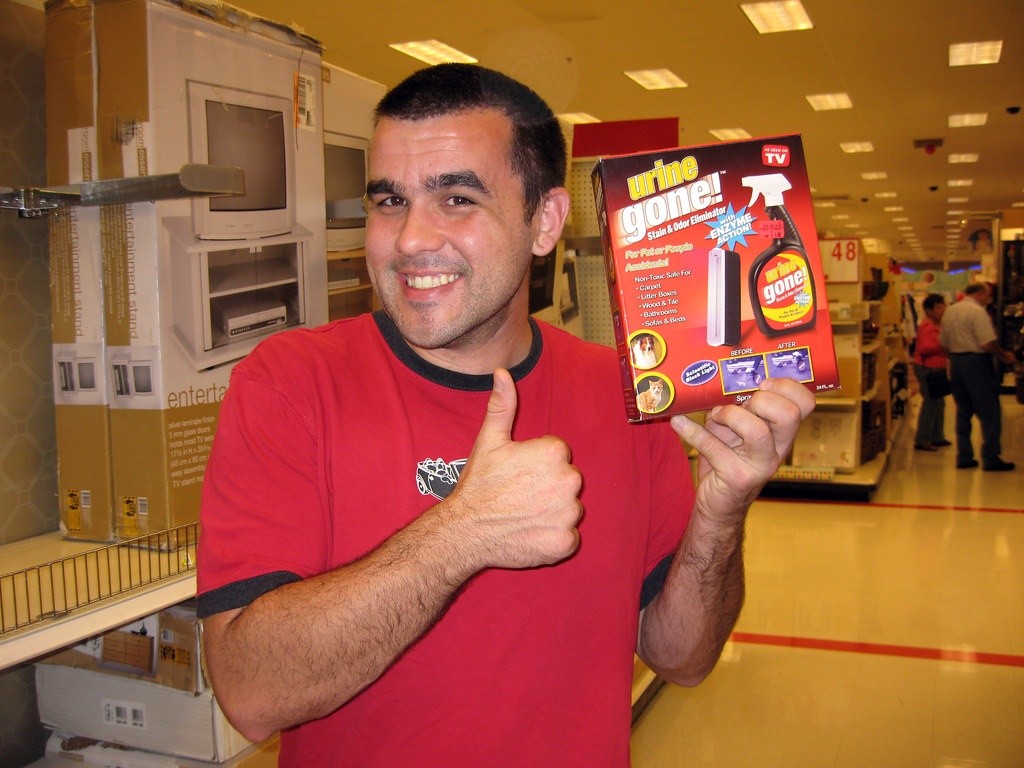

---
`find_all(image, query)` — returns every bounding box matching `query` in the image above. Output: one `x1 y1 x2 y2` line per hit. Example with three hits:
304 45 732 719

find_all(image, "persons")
913 294 951 452
195 63 816 768
938 282 1016 471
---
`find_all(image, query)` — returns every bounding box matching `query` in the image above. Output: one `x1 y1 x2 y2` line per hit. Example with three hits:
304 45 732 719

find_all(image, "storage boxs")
588 131 844 425
33 0 392 767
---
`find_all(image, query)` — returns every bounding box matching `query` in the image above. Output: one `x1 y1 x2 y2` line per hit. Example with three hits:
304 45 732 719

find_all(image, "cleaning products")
741 173 817 338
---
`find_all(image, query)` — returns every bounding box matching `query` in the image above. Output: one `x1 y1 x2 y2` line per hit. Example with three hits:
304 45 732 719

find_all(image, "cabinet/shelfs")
766 238 910 502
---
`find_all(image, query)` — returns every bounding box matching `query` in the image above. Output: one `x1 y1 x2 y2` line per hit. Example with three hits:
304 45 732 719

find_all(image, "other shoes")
957 459 979 468
931 438 951 447
915 441 938 451
982 457 1015 472
